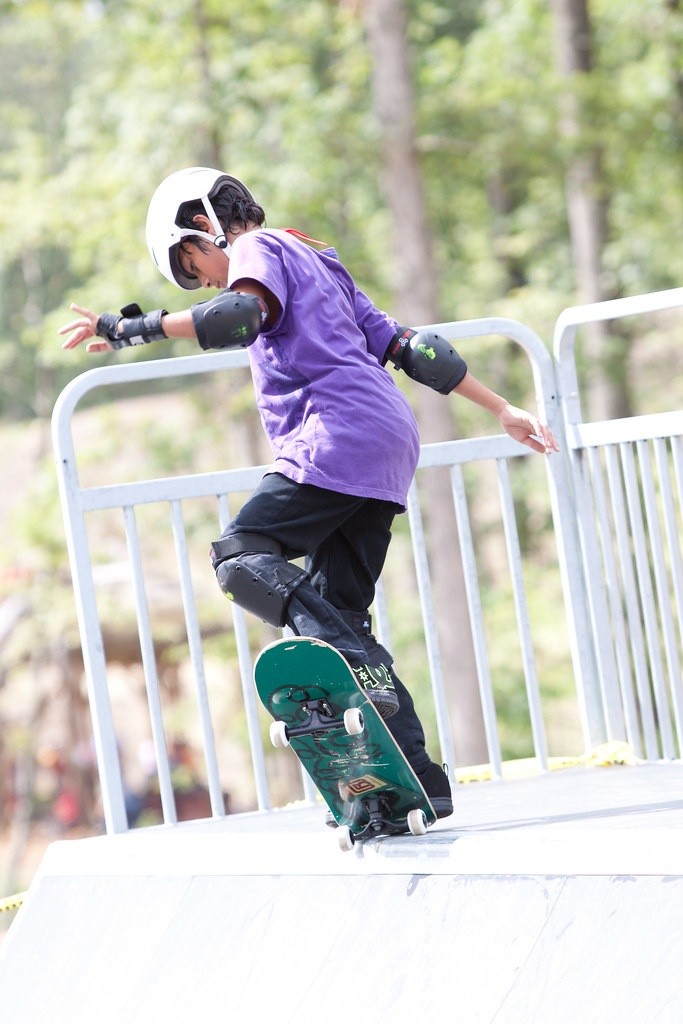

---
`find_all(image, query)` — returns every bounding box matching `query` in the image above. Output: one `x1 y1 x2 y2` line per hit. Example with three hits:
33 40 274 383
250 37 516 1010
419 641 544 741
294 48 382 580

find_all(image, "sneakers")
350 662 401 721
419 765 452 820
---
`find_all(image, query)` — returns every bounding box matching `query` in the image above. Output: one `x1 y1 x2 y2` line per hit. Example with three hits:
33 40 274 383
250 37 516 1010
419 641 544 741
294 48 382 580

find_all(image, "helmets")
144 169 255 290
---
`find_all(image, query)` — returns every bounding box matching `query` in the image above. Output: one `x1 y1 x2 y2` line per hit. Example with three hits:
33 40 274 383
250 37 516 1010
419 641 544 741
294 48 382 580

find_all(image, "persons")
60 167 563 821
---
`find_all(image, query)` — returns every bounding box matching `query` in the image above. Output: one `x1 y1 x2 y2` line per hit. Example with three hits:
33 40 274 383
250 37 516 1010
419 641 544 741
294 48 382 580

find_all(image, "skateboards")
250 634 437 841
